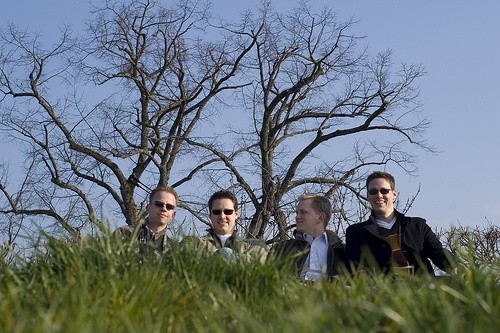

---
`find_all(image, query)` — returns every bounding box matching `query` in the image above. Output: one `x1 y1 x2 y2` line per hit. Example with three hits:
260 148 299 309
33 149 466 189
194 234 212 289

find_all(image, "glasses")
211 209 234 215
367 189 391 195
151 201 174 210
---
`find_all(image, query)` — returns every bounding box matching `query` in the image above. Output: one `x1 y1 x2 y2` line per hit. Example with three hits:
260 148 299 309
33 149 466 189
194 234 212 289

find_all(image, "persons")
265 193 346 287
112 185 185 266
346 171 457 279
193 190 269 268
